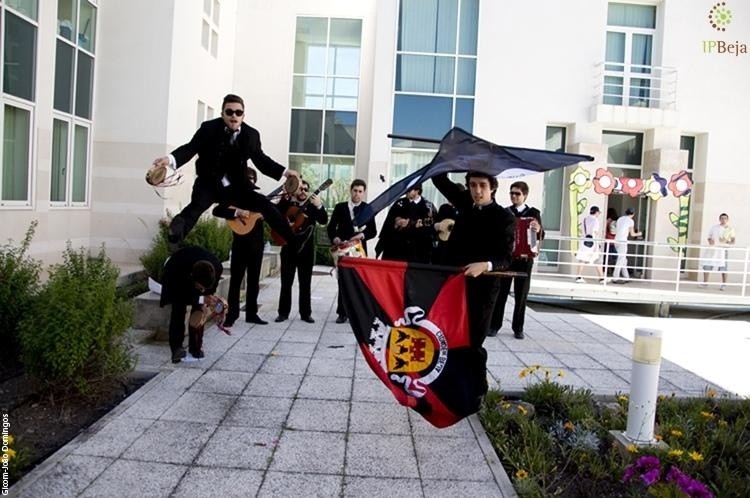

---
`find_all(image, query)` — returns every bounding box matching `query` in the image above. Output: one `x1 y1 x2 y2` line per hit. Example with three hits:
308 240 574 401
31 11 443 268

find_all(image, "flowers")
567 167 697 259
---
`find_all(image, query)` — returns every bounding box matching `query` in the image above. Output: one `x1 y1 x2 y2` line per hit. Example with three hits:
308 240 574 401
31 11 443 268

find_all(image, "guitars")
227 185 283 234
271 178 333 246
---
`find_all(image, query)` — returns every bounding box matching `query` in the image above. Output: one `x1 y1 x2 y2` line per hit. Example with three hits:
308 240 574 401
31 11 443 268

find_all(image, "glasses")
223 108 244 117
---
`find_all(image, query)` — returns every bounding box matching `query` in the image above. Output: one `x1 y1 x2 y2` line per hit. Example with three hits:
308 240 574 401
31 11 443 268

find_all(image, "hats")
590 206 601 214
627 208 636 213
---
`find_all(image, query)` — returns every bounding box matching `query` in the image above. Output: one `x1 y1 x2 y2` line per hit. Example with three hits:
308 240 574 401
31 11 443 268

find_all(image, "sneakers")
612 277 634 284
698 283 709 289
599 278 610 284
720 285 727 291
576 277 585 283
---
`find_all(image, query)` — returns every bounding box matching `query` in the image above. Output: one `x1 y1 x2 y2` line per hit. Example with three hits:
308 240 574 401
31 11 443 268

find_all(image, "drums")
329 238 366 279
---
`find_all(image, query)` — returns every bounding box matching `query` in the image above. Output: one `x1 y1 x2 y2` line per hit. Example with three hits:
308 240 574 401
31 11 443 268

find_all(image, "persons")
612 207 643 283
606 207 618 273
574 205 611 283
373 181 437 264
487 180 546 338
432 171 518 345
274 179 328 322
154 94 314 254
212 165 269 327
327 177 377 323
698 212 736 290
160 245 224 362
432 182 467 252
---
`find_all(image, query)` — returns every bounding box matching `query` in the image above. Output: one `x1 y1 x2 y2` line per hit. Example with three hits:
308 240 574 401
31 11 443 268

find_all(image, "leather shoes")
292 225 314 256
223 312 239 327
512 331 524 339
336 314 347 323
245 313 268 324
168 215 185 244
300 314 314 323
275 313 288 322
189 345 204 358
487 327 502 336
172 347 187 364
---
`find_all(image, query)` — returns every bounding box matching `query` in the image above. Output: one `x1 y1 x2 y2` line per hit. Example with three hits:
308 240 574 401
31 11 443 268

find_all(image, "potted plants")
128 209 283 341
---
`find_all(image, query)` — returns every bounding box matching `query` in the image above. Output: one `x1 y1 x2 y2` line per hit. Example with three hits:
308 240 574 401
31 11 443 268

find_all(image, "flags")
350 126 596 229
337 254 487 427
